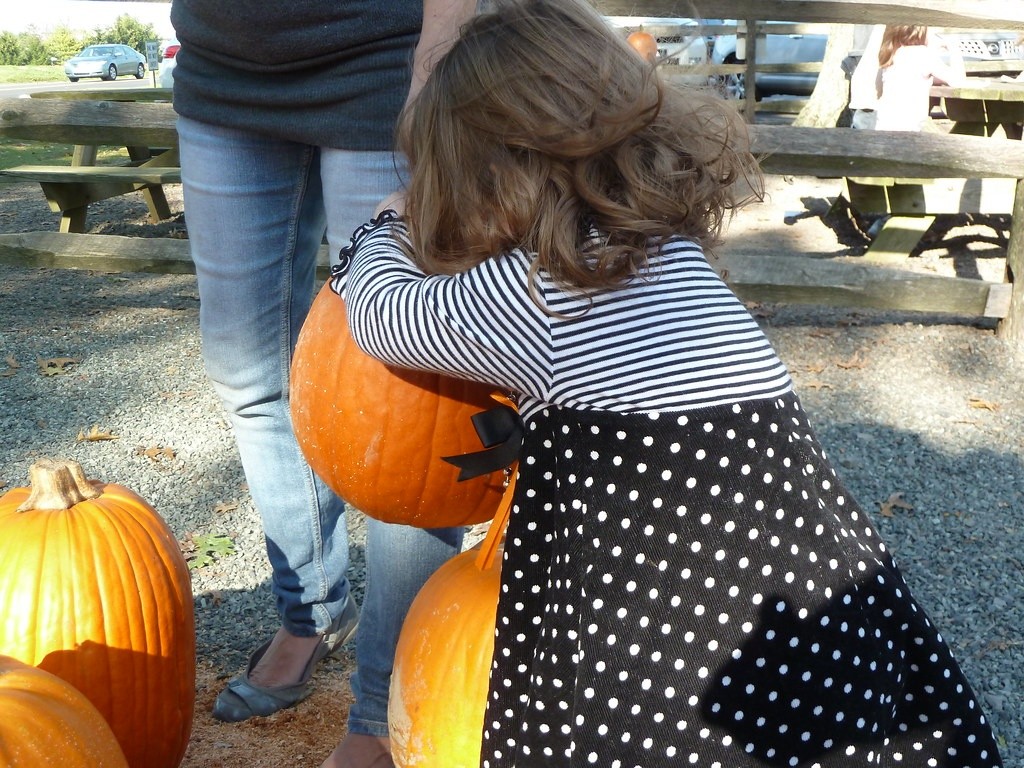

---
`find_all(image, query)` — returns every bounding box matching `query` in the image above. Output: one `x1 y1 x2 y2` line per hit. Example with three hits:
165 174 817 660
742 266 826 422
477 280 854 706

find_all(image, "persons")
342 1 1010 768
166 0 485 768
841 22 969 135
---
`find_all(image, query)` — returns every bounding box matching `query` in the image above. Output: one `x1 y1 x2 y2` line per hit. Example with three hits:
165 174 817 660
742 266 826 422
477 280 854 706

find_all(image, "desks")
30 88 178 234
863 84 1024 261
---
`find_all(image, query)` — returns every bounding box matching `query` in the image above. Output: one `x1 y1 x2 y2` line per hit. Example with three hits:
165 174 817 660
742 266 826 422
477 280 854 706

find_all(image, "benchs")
0 165 179 234
849 176 934 188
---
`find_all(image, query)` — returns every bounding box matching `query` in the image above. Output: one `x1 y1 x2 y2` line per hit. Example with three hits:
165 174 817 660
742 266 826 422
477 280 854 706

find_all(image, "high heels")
212 595 363 723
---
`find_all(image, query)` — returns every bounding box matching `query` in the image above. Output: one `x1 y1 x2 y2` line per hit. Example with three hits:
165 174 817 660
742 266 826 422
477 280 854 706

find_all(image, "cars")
158 40 181 88
706 19 1024 116
598 16 708 95
64 44 146 82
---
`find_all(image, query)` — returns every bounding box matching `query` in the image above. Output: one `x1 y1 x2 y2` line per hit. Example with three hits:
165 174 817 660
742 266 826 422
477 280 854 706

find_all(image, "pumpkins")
388 451 518 768
0 461 197 768
289 275 523 527
